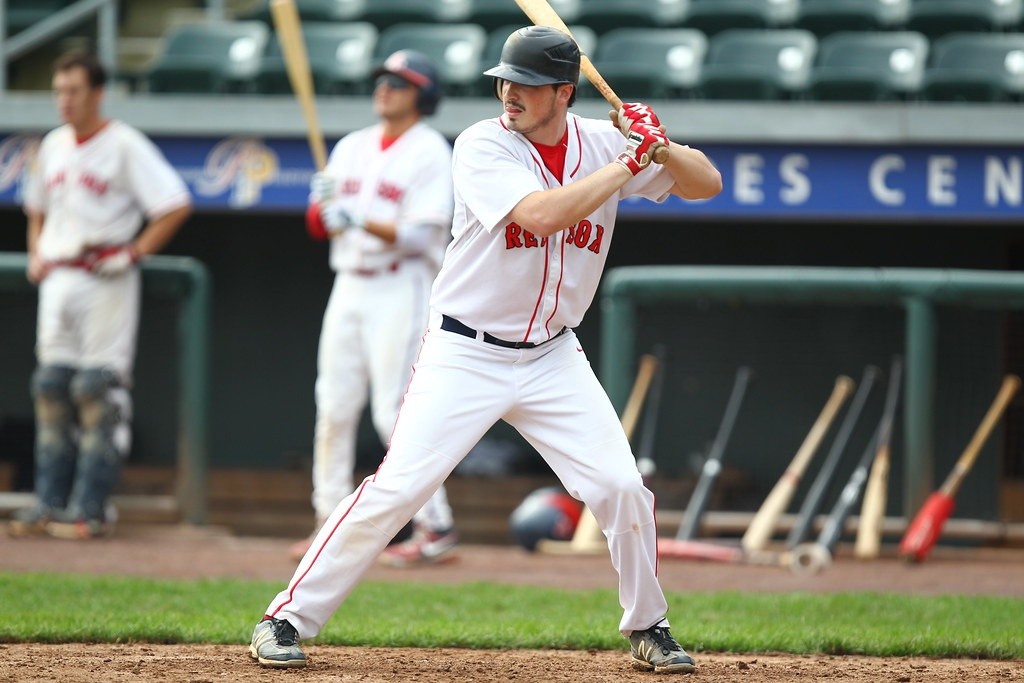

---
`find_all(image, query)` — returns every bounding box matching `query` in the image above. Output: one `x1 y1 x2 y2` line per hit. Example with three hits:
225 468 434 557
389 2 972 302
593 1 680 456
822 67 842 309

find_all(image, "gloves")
308 165 340 203
319 200 362 232
609 102 661 139
615 123 669 176
90 245 137 278
24 245 49 282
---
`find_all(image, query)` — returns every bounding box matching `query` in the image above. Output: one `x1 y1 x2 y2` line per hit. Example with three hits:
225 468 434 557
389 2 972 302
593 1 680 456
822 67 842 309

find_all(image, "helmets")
482 26 581 102
369 48 439 114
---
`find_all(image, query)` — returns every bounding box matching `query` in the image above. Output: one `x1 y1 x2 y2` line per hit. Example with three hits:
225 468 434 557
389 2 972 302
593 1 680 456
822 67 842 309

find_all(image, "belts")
441 314 567 349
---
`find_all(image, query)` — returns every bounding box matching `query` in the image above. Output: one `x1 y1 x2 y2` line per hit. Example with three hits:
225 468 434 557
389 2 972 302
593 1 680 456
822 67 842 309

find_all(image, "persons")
9 51 193 540
287 48 458 568
250 25 722 676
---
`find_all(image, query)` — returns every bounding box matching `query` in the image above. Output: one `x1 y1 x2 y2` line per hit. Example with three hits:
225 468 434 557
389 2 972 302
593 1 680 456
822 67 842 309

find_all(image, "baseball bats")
861 356 900 554
785 365 875 552
657 537 796 567
675 365 753 542
573 353 657 546
740 375 854 546
516 0 671 166
268 0 341 239
901 375 1015 563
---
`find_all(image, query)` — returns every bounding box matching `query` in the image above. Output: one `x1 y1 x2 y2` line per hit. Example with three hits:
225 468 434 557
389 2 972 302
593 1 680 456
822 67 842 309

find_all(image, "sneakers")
7 494 118 538
629 626 695 673
248 616 306 668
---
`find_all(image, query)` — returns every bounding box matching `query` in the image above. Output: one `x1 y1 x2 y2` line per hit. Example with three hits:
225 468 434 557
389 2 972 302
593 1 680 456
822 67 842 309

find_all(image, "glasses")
373 72 409 90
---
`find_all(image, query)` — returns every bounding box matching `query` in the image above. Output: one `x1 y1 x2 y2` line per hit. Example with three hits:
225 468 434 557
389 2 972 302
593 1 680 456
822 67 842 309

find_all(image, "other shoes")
375 527 454 566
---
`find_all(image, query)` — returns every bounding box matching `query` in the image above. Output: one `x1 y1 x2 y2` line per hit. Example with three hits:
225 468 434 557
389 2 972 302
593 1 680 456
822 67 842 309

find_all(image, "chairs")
917 33 1024 105
690 30 821 99
234 21 379 95
805 31 932 103
480 24 598 100
588 28 708 99
134 18 268 92
368 24 487 97
